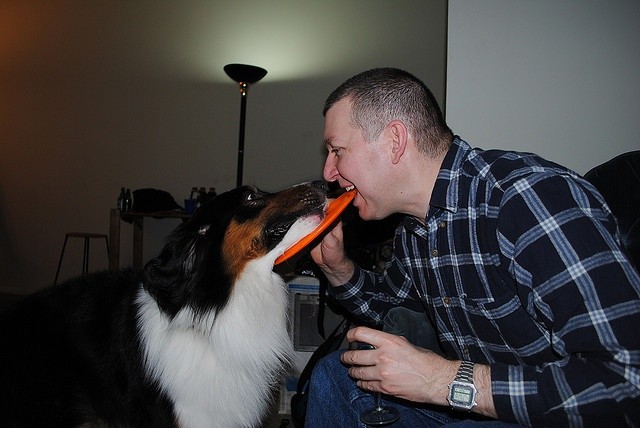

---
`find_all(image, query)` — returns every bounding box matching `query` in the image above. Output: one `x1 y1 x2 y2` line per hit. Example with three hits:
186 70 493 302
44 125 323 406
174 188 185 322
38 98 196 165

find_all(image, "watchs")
446 360 477 413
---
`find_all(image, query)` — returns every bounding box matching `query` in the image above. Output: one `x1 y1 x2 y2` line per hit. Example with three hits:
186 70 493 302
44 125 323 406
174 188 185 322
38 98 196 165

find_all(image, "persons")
307 67 640 428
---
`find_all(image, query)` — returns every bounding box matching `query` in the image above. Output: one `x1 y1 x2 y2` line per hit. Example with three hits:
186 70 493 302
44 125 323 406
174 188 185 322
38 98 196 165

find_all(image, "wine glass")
347 322 400 426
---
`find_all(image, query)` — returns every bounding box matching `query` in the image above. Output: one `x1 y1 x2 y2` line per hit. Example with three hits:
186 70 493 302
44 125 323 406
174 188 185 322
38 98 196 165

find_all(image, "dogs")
0 179 329 428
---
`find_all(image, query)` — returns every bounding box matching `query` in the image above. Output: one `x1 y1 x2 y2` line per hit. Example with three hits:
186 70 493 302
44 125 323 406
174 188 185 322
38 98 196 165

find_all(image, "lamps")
222 63 267 188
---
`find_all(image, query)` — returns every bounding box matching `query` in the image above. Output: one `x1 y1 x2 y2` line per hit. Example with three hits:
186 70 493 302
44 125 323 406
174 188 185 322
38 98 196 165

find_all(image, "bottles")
191 187 199 209
118 187 125 214
126 188 132 214
208 187 218 202
200 186 206 206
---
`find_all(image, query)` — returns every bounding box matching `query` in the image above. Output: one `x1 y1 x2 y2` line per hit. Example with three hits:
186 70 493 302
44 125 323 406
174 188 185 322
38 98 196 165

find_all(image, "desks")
109 209 202 281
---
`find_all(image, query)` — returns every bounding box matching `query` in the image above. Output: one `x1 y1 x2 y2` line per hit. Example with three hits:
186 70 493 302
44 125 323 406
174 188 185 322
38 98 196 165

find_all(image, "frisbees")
274 188 357 266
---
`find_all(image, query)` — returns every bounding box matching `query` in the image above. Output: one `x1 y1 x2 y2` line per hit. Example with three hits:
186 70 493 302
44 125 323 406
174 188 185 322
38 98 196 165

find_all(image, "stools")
53 231 108 292
